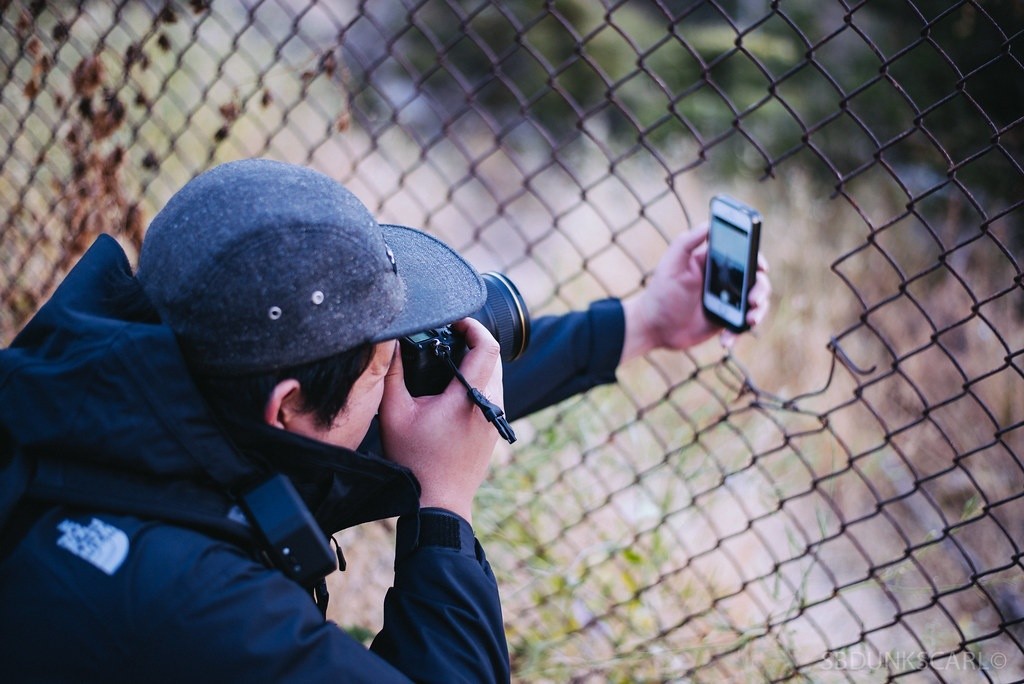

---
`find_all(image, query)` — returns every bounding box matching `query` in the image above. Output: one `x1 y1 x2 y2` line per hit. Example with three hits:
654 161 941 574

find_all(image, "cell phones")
702 193 761 331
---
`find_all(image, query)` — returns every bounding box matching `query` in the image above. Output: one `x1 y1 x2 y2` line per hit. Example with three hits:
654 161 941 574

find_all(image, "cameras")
400 272 531 398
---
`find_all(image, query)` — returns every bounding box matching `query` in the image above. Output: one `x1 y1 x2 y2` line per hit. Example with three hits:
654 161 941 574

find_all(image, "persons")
0 161 774 683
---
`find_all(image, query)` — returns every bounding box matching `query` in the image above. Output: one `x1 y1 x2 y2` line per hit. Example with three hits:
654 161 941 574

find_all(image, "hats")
135 159 487 377
